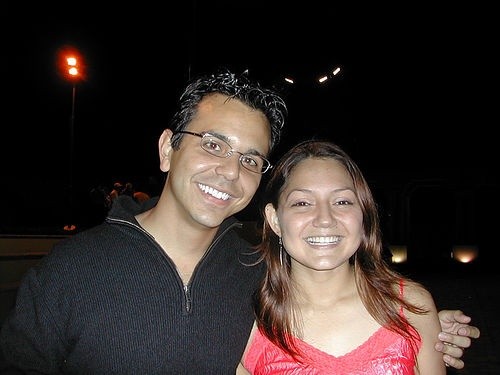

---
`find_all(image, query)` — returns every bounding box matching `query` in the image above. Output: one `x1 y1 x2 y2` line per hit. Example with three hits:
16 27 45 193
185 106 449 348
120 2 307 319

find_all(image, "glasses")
174 131 273 174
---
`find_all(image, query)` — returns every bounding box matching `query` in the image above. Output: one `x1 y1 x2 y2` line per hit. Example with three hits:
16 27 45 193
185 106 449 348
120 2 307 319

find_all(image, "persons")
0 74 480 371
237 141 447 375
